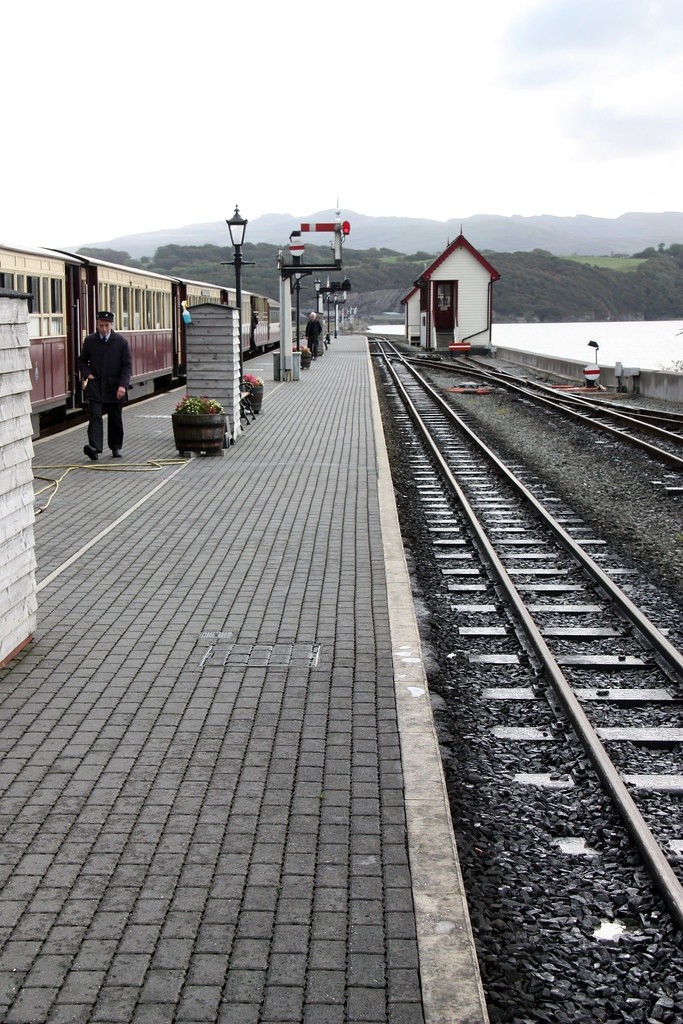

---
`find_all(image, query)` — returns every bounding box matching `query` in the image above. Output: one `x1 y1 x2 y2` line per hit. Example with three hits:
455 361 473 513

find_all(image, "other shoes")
113 448 121 457
314 356 317 360
84 444 99 460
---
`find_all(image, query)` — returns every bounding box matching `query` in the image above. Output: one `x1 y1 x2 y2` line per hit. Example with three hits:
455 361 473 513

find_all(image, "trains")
0 243 298 440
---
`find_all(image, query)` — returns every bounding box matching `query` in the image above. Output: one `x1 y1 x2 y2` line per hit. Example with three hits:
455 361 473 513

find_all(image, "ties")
102 336 106 344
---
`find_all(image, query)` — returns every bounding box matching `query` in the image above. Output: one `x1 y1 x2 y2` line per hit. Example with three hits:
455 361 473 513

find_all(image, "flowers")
175 393 224 413
293 345 311 353
244 374 263 387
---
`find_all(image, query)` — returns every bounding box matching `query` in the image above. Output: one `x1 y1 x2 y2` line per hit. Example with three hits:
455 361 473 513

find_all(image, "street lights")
313 276 322 315
226 203 248 392
333 293 338 340
295 272 303 352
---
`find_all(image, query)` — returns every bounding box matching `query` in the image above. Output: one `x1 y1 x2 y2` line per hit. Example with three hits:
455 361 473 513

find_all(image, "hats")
98 312 113 322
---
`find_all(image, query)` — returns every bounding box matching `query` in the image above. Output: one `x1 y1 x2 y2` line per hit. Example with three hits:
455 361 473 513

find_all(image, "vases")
301 353 313 368
171 413 225 450
242 387 263 410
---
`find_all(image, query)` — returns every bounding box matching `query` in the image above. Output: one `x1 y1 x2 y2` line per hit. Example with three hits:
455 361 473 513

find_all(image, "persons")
80 312 132 461
249 309 258 351
305 313 321 360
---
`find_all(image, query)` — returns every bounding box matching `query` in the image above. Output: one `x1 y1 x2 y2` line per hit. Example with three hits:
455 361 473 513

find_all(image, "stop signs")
585 364 601 382
